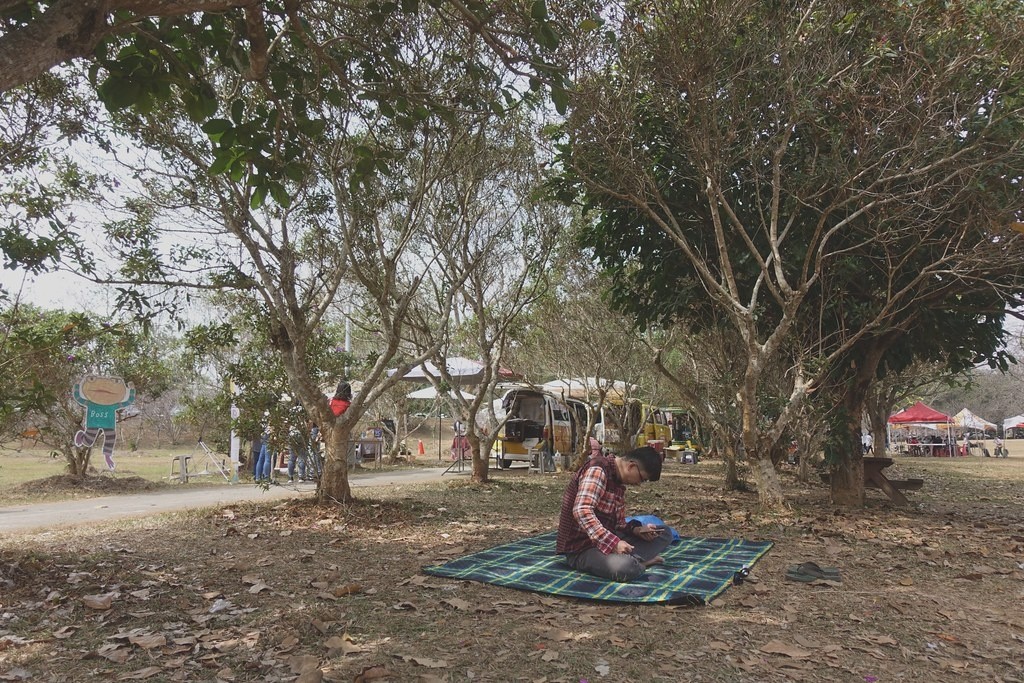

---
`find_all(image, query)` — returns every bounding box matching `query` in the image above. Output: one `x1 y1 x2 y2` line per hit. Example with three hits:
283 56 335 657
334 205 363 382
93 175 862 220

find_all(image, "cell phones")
640 529 665 533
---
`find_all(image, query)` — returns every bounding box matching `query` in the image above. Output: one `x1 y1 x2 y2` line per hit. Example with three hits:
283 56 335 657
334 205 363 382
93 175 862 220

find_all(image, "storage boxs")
648 439 665 452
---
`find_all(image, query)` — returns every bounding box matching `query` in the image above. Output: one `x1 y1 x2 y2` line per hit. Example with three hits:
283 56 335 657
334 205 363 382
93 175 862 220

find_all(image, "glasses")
630 460 648 482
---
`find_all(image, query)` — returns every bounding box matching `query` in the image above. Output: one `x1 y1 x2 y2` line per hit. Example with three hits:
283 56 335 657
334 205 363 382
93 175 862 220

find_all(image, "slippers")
783 561 843 583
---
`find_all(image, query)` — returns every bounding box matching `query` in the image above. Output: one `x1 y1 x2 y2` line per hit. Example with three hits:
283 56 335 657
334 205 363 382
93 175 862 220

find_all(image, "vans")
492 383 725 472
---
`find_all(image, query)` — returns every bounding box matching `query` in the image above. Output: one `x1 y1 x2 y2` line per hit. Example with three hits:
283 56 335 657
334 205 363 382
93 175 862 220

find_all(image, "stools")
528 449 549 475
171 455 200 485
682 452 698 464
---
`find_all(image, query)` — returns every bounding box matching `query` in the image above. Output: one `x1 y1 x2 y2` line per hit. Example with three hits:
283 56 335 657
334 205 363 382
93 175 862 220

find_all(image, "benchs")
907 478 924 490
819 474 830 485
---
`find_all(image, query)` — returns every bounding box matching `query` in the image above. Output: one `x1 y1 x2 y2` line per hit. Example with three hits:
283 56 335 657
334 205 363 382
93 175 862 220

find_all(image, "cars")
965 430 1024 439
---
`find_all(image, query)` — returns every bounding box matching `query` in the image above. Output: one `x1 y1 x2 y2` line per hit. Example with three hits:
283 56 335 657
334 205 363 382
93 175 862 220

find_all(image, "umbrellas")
404 385 479 464
385 355 524 478
540 377 639 401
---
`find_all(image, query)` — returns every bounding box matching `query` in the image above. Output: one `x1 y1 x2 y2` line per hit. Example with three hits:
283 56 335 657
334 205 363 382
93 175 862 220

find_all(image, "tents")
944 407 997 445
887 404 962 460
891 406 961 448
1001 412 1024 454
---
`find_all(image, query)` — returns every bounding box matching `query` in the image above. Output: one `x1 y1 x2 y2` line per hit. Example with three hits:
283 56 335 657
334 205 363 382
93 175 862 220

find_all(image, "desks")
863 457 908 507
907 443 946 456
347 438 386 471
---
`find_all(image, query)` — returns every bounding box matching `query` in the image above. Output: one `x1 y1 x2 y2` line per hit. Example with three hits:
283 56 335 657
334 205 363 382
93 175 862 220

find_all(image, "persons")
556 445 673 582
860 429 1024 456
252 400 325 485
